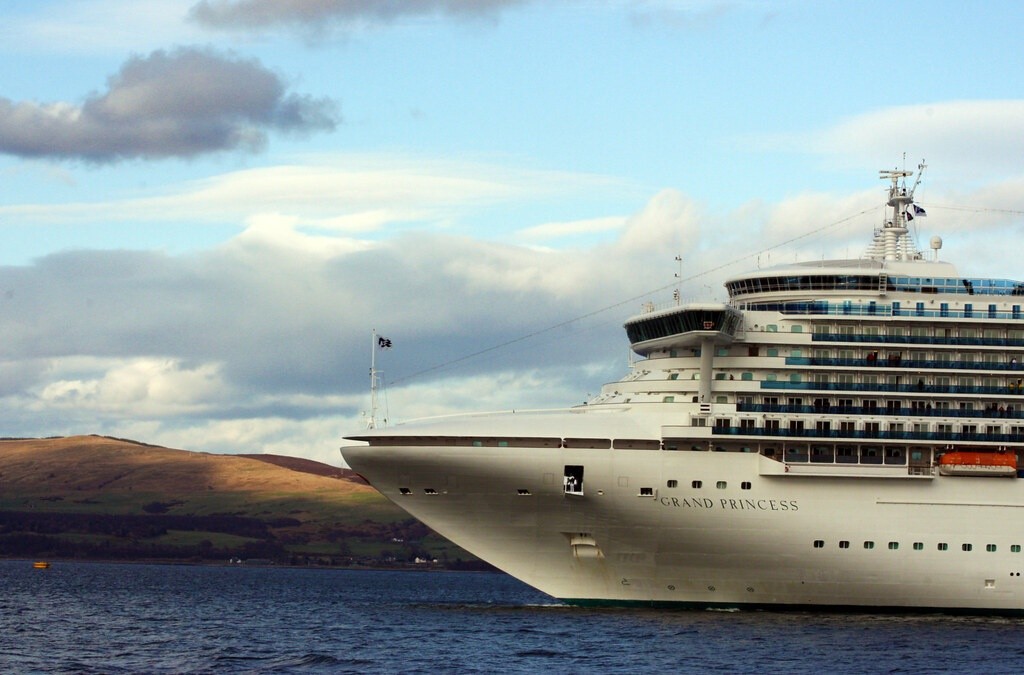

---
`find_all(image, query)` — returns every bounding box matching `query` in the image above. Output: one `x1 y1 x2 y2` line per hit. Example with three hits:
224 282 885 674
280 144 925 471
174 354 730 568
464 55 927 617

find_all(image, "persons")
910 403 938 415
985 404 1013 417
1012 283 1024 295
1010 358 1017 364
866 353 901 366
1008 382 1022 394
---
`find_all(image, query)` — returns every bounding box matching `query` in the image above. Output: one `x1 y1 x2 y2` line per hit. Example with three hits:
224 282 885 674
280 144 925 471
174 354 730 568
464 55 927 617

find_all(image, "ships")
340 149 1024 611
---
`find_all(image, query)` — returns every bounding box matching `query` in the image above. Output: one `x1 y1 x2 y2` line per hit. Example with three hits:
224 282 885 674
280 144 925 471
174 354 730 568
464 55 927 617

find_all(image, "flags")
378 337 392 349
907 212 913 221
914 204 926 216
